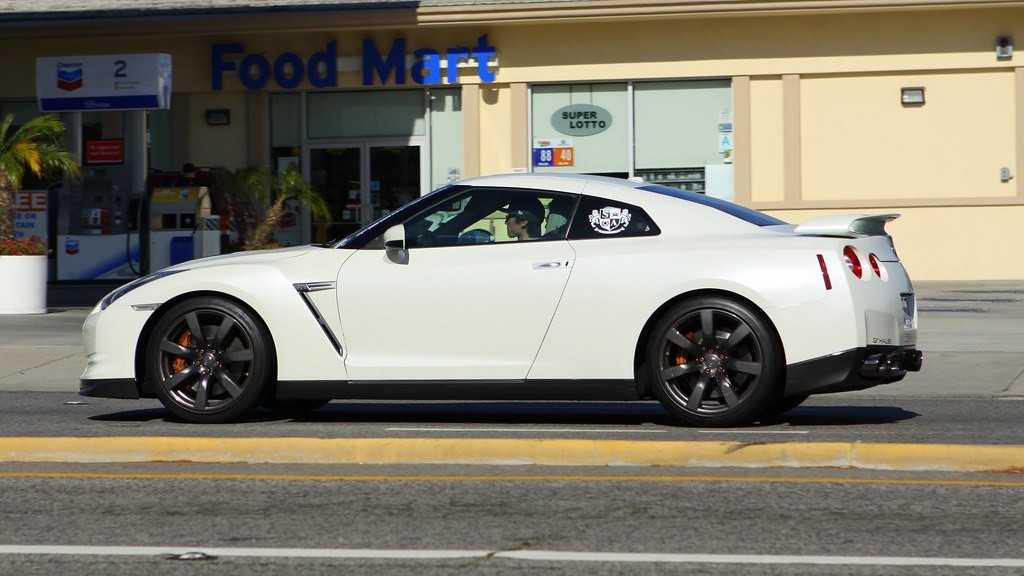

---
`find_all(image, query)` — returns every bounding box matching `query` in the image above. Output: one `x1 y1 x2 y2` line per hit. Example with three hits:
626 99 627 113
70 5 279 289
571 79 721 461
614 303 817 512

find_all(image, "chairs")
542 196 577 242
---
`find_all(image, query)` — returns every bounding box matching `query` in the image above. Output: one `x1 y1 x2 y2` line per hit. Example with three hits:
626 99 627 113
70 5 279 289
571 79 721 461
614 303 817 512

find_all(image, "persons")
161 176 177 228
503 195 546 240
177 162 206 228
543 201 574 240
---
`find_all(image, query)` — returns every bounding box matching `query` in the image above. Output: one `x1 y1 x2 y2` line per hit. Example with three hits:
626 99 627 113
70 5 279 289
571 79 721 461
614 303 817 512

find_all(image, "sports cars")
77 171 925 429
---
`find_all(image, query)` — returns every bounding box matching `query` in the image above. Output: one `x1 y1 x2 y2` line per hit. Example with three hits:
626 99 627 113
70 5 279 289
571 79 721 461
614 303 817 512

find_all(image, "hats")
497 196 546 222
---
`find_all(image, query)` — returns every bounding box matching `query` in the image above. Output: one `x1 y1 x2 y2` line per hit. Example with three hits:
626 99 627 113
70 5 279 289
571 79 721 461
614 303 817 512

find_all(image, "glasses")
506 215 515 221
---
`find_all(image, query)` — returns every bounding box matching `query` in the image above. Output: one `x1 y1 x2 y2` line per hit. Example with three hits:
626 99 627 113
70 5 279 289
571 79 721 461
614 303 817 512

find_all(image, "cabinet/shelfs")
633 167 706 195
